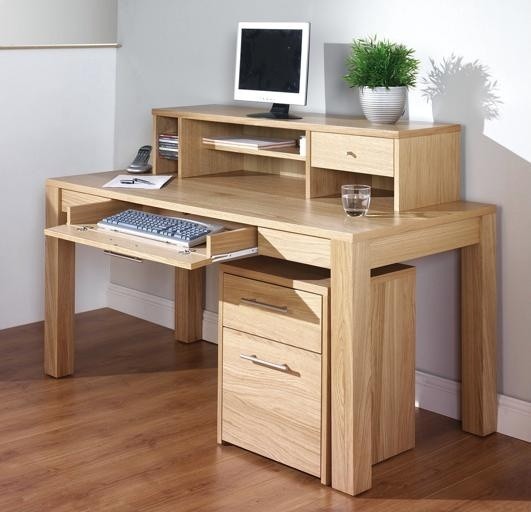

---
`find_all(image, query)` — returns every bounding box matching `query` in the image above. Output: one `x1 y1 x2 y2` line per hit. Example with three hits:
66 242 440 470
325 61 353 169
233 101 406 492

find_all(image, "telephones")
126 145 152 173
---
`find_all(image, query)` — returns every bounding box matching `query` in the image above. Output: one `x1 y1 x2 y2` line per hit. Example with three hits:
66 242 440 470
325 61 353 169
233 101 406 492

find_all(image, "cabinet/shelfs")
217 257 417 487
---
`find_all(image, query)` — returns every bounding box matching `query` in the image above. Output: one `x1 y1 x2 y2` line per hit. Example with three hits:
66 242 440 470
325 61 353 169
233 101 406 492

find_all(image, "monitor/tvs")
233 21 311 120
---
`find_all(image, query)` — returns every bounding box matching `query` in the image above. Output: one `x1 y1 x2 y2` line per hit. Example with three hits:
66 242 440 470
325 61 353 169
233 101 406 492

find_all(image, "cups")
340 183 373 220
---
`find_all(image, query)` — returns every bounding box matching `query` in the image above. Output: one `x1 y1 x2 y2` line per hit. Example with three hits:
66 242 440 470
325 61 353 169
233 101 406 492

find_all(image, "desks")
43 105 498 497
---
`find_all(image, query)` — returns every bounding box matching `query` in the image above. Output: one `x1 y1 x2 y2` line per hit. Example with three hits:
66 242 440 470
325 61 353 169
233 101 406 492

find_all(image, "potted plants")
341 35 420 125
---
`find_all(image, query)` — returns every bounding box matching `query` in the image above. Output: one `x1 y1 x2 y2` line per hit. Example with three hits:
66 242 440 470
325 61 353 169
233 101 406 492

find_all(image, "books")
202 135 298 150
157 133 179 159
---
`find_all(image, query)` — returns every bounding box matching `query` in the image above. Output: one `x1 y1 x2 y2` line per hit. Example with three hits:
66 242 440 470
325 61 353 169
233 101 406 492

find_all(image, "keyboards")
97 208 225 249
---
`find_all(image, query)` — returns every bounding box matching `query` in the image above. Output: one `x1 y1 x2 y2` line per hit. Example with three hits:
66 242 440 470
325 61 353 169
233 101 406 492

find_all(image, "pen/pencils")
121 178 155 185
